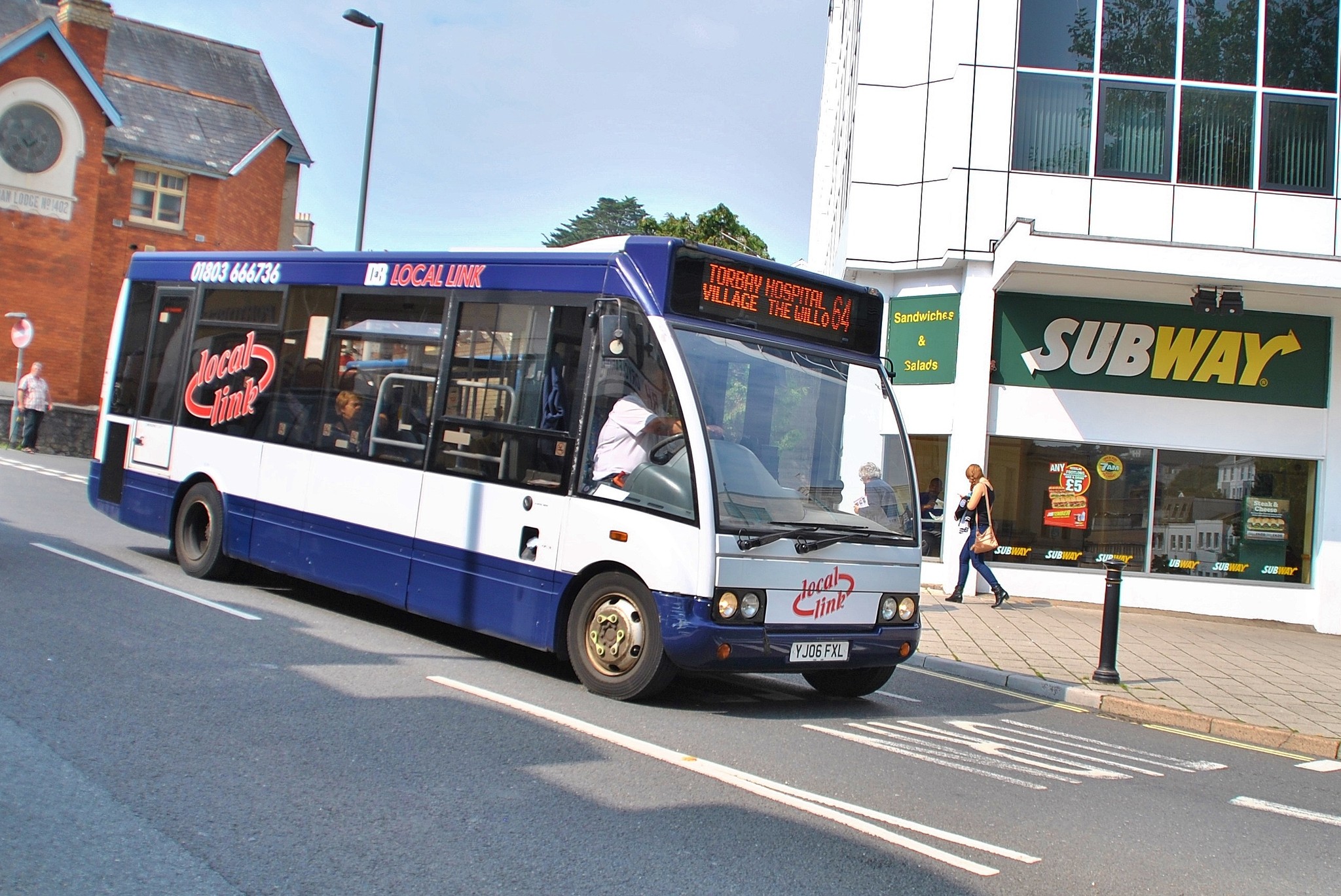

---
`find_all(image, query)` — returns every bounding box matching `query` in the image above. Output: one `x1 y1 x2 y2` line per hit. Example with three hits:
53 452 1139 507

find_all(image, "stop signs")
10 319 33 349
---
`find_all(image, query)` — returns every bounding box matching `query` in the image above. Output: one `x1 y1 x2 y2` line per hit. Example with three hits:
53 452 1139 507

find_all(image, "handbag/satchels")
974 528 999 555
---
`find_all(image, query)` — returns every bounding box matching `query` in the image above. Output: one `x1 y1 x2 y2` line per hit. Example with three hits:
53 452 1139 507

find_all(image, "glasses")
860 478 865 481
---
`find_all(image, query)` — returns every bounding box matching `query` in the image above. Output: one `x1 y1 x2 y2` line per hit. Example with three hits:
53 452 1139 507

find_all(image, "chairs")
275 403 564 489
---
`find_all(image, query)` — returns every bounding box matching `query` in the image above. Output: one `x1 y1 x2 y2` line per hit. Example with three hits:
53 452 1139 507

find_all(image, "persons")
332 391 373 454
945 464 1009 608
853 462 901 533
18 362 54 454
919 478 944 556
591 359 726 484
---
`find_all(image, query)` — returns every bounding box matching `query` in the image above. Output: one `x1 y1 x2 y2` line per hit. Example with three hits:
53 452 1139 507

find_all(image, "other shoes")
22 446 38 454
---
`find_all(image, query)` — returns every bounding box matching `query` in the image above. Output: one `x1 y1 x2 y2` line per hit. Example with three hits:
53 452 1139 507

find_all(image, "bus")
86 232 923 705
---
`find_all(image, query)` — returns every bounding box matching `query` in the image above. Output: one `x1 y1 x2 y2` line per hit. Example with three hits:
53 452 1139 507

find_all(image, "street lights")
340 4 385 254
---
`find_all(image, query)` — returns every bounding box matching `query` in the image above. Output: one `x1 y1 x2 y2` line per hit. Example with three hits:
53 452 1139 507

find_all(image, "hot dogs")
1048 486 1086 509
1246 517 1285 531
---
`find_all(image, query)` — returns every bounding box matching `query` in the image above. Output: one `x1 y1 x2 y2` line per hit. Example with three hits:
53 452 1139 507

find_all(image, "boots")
946 586 965 603
991 585 1010 608
